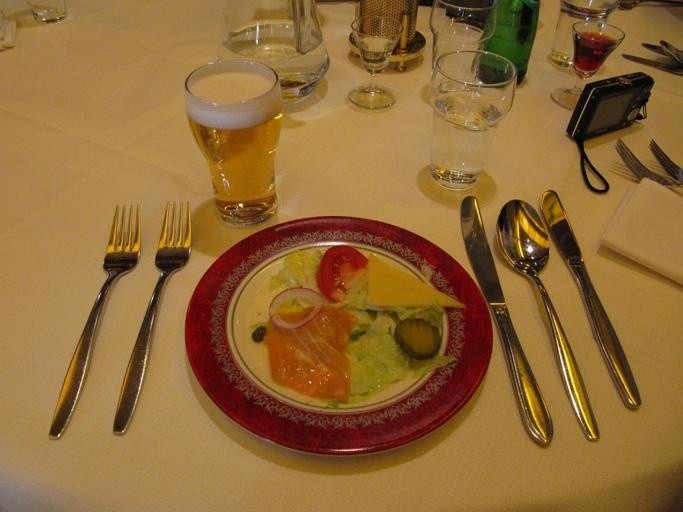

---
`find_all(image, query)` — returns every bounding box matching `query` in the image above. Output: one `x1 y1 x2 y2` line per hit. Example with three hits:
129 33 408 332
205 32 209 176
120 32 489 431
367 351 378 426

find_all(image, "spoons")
495 197 601 446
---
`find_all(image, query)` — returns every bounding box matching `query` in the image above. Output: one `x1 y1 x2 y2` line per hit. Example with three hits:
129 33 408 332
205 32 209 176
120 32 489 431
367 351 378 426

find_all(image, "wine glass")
343 15 405 111
550 19 627 112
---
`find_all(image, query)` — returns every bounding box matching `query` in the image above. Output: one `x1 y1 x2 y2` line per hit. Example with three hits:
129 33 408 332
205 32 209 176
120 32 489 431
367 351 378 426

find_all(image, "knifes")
458 193 554 448
538 187 642 411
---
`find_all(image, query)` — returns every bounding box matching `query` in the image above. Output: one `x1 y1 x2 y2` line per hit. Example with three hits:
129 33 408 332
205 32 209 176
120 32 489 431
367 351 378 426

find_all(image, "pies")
365 253 466 310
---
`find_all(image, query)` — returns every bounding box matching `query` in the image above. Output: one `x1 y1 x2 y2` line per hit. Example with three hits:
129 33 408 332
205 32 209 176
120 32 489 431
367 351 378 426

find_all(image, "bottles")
471 0 541 89
216 1 331 104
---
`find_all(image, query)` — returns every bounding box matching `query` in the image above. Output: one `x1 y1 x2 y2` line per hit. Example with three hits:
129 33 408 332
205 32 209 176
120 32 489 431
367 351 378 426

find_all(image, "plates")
182 212 495 462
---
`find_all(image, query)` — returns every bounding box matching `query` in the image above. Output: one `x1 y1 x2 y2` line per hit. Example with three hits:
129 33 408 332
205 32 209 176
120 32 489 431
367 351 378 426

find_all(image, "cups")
184 57 284 230
425 46 519 193
546 0 623 73
429 1 498 94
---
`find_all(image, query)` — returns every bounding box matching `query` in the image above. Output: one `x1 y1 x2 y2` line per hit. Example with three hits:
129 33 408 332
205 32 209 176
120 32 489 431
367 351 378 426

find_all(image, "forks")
613 134 683 191
47 202 143 443
112 200 194 438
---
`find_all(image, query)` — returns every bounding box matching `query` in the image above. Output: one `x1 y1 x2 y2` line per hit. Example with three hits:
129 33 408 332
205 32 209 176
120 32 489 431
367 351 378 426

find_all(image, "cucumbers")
395 316 441 359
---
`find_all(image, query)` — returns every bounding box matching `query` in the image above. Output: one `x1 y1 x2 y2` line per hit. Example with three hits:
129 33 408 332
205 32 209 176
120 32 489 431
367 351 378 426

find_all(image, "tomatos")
317 240 369 306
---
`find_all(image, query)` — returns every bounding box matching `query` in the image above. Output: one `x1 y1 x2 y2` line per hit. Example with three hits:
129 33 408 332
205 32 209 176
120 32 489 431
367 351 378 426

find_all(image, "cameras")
565 70 655 143
